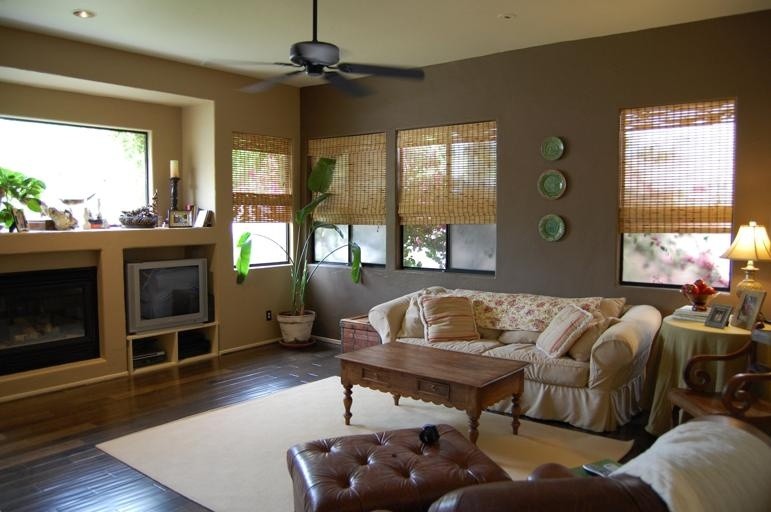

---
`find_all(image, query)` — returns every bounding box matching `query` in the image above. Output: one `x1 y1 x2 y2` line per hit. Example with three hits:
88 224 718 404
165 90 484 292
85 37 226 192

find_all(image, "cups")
45 220 55 230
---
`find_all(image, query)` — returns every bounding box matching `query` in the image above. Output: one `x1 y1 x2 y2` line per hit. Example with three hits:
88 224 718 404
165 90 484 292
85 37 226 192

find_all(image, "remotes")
582 464 610 478
604 464 621 472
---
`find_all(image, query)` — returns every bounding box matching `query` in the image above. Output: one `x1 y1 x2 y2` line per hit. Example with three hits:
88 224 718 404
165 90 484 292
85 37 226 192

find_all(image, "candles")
170 159 179 178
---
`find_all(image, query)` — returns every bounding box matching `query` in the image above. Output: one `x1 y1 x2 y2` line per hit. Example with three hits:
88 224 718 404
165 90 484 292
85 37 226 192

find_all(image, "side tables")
645 314 771 436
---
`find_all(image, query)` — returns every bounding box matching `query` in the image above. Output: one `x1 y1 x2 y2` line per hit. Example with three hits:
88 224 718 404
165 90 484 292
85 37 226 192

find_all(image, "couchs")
429 422 770 512
368 285 663 435
286 424 512 512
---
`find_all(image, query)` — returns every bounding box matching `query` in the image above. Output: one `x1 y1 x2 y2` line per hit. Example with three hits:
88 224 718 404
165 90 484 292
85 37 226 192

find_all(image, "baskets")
119 215 158 228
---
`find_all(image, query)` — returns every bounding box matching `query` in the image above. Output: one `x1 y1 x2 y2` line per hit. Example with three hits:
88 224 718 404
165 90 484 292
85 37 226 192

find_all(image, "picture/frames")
168 210 193 228
731 289 767 331
705 304 733 329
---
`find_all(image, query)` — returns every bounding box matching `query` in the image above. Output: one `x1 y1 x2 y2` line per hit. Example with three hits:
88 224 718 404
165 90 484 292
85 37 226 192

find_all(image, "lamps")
719 220 771 297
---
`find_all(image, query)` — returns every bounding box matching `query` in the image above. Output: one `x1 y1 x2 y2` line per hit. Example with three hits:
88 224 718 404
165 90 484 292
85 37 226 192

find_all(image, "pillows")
569 316 623 361
397 289 436 338
596 296 625 315
496 329 540 344
536 303 593 358
418 295 480 343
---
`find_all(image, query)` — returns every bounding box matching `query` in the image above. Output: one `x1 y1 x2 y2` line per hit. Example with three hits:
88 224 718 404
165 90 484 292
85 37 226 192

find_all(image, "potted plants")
235 157 362 348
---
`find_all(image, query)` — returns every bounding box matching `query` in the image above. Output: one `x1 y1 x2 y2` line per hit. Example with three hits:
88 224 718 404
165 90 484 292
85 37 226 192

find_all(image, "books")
671 304 712 323
582 457 623 478
184 204 212 227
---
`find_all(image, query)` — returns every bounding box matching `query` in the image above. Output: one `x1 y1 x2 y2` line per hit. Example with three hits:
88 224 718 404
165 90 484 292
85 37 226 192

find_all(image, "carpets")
95 376 635 512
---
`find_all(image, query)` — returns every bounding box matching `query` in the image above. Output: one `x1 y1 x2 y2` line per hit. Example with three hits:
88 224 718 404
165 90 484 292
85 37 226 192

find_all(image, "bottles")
83 208 89 229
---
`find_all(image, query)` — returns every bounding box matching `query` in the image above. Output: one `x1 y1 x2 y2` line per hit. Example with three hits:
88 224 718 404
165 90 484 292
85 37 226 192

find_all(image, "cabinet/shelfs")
127 321 219 375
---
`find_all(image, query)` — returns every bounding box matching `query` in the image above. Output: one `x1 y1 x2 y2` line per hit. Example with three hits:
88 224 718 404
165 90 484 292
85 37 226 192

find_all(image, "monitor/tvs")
127 258 209 335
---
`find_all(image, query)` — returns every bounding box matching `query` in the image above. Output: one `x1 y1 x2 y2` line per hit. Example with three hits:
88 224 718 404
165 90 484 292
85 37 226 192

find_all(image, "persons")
714 310 722 323
174 211 180 223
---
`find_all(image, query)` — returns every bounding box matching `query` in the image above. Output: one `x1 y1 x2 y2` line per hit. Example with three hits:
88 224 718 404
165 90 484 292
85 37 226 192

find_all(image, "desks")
339 315 381 352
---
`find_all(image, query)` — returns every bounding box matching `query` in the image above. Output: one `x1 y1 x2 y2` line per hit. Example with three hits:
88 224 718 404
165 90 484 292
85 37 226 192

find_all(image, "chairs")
666 321 771 429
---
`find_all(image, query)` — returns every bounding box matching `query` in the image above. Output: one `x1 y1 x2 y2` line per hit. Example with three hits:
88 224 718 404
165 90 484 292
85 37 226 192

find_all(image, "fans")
208 1 425 98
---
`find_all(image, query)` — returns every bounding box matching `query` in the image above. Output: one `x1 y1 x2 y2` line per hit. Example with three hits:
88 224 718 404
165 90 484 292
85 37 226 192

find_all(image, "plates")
538 214 566 241
537 170 567 200
539 136 565 160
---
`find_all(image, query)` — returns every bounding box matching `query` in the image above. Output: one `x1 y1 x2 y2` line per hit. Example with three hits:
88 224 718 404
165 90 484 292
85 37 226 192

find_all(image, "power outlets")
265 309 272 320
